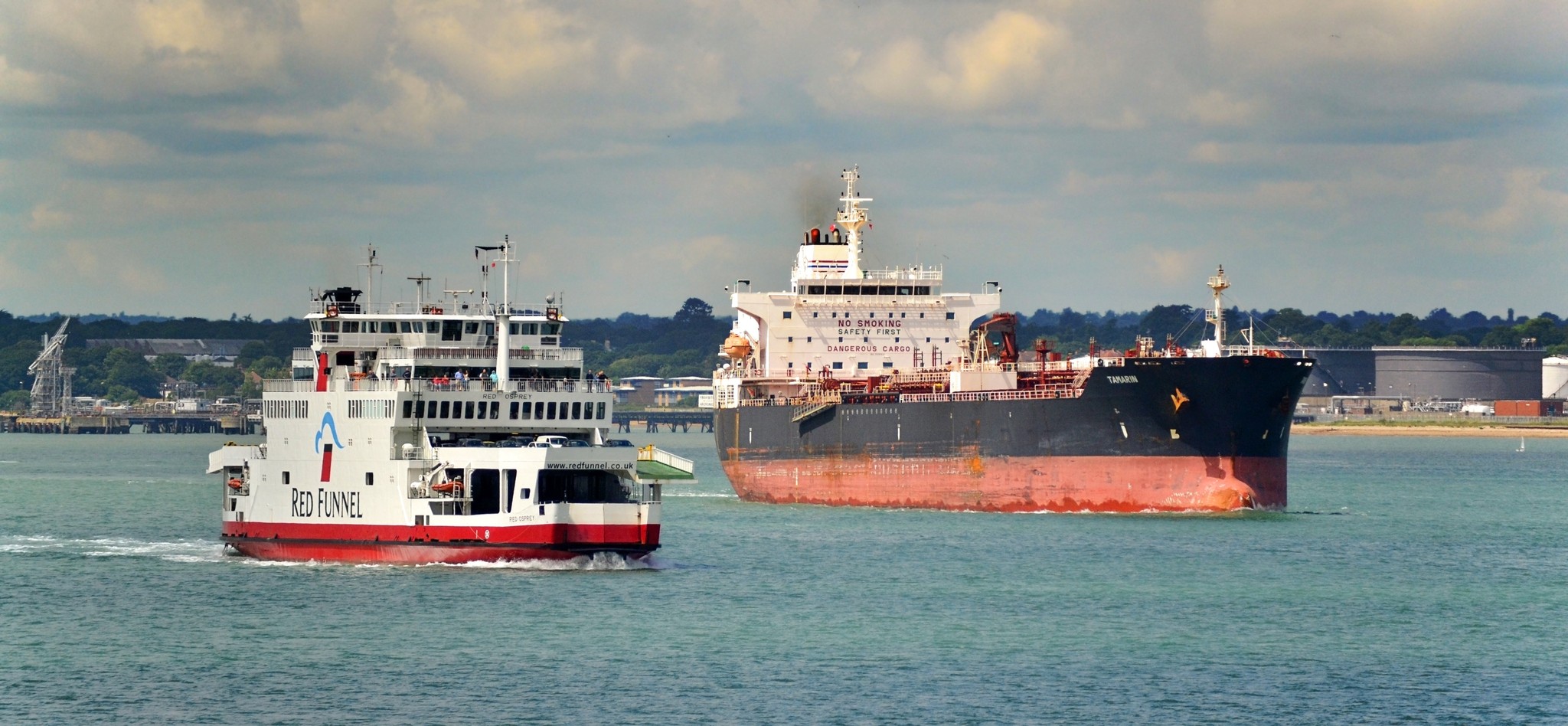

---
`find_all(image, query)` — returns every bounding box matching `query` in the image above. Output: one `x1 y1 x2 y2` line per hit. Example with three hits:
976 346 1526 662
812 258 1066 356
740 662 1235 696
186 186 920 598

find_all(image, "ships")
712 162 1317 516
205 232 663 566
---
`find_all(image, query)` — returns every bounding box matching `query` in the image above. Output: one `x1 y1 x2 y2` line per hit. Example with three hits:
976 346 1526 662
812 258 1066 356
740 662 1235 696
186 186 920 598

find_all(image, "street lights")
176 384 179 401
163 381 167 402
20 381 23 418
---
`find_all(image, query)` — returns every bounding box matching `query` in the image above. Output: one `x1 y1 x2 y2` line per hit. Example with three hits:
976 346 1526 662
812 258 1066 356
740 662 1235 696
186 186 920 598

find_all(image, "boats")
432 481 464 493
227 478 242 489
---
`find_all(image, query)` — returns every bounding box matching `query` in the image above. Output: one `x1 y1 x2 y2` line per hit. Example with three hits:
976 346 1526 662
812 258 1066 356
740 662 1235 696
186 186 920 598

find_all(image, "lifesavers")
755 370 762 376
547 312 556 320
328 309 338 317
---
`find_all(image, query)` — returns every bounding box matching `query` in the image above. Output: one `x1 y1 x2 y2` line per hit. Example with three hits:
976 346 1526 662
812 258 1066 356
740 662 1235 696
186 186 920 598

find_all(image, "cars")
434 436 634 449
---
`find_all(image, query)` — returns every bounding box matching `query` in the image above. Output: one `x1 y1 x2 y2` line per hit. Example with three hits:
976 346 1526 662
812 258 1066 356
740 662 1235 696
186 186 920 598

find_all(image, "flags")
830 224 835 232
869 224 872 229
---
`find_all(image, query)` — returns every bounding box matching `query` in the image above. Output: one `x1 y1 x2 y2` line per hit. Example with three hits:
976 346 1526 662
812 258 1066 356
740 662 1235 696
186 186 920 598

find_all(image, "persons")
350 369 610 393
